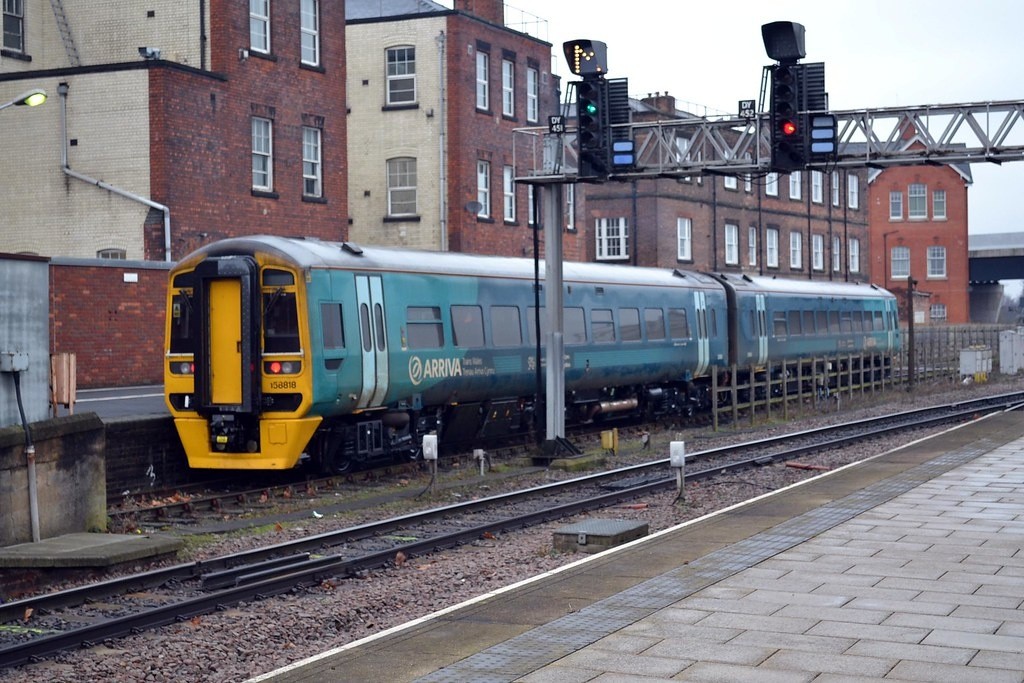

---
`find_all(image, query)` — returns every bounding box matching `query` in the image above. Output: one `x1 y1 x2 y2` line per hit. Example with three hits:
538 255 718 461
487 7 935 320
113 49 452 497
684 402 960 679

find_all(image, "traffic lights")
609 137 636 171
762 21 806 59
564 40 608 75
769 63 805 174
810 113 837 162
577 81 604 183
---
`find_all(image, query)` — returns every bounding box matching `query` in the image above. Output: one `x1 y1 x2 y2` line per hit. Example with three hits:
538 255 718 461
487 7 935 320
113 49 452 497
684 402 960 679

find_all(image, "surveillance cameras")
139 46 154 57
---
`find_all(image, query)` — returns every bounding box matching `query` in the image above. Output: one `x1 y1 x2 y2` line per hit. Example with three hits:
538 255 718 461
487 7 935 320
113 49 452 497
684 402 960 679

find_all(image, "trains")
165 235 903 473
